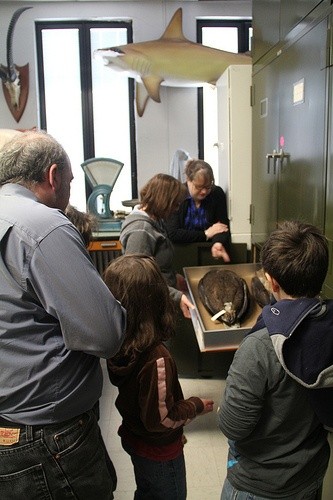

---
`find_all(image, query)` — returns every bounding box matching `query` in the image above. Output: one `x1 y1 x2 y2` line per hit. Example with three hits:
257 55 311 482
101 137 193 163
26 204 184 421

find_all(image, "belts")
0 422 40 433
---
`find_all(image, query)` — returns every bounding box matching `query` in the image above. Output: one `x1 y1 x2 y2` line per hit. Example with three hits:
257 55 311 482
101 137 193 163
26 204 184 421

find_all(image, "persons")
170 160 232 263
102 254 214 500
119 173 222 378
217 220 333 500
0 129 130 500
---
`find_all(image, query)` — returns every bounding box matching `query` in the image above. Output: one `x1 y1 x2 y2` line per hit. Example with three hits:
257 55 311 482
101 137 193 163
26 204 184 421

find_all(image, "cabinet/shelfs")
182 0 333 380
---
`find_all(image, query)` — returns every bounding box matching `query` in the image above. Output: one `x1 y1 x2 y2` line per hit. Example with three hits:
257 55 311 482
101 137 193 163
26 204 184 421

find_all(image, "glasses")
191 178 215 190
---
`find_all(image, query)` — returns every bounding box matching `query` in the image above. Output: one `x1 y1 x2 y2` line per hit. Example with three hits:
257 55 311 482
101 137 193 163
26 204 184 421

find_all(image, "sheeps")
0 6 33 111
